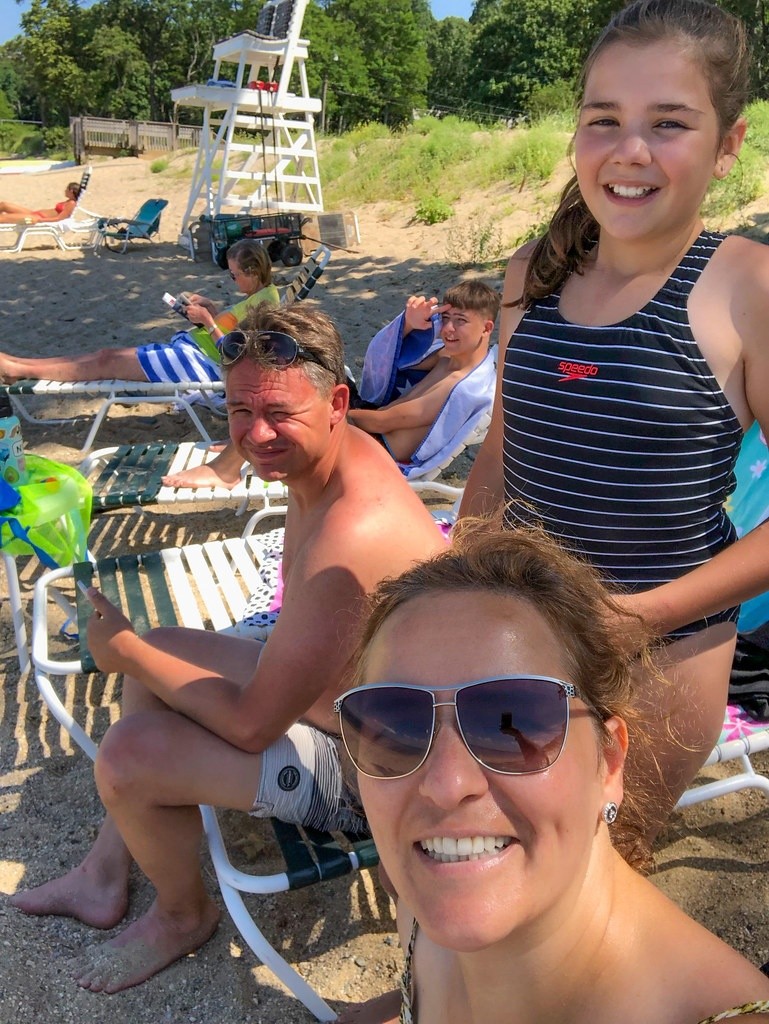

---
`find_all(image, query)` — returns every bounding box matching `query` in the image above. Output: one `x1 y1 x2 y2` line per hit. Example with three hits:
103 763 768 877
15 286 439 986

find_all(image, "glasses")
221 330 326 371
333 667 583 776
229 271 236 280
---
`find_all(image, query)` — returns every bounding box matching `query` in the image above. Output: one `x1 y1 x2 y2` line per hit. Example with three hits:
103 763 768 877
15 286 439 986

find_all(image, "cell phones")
76 580 89 600
179 293 192 306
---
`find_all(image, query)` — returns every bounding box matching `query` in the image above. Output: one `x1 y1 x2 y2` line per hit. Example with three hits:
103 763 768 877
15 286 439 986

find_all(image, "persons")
159 280 500 491
332 529 769 1024
449 1 769 873
724 417 769 720
0 182 80 225
8 304 459 994
0 238 280 383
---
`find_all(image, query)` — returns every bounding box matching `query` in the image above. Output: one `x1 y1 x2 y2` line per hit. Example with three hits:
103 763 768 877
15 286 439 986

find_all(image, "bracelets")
208 323 217 336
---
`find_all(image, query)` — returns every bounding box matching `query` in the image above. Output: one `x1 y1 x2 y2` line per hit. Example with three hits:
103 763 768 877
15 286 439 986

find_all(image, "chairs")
96 198 169 254
0 246 769 1024
0 166 104 253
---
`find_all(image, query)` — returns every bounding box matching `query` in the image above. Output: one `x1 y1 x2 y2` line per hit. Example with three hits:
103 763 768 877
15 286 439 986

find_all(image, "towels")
358 307 499 475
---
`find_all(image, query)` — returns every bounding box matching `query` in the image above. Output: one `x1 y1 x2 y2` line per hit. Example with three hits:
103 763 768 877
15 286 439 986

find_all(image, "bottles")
0 386 28 487
